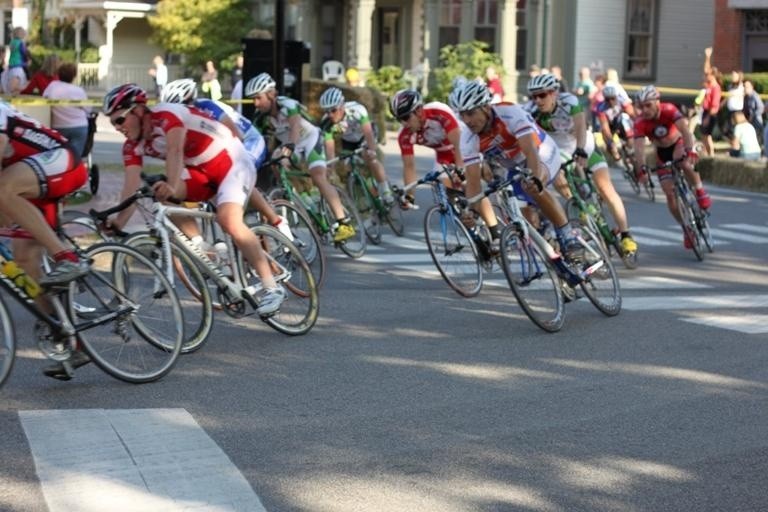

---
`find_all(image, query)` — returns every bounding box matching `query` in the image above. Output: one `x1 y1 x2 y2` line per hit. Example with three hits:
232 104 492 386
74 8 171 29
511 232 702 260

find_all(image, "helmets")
320 87 345 109
161 78 196 104
103 84 148 115
603 87 618 97
635 85 660 101
245 73 276 97
527 75 560 93
392 90 422 117
449 82 490 111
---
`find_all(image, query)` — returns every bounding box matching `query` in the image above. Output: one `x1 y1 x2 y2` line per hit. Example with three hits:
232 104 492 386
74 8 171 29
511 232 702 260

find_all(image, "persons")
244 70 356 243
588 75 607 133
603 68 628 97
758 98 768 164
41 62 95 157
523 63 568 102
0 99 93 377
632 84 712 249
0 54 32 95
742 79 765 131
147 55 168 103
20 53 65 98
388 87 504 252
199 60 218 100
316 87 395 231
8 25 28 69
699 46 722 157
474 67 506 105
158 77 295 244
571 66 595 131
726 111 762 161
522 73 639 260
595 86 642 162
229 55 245 89
96 82 290 319
447 80 582 253
718 67 746 145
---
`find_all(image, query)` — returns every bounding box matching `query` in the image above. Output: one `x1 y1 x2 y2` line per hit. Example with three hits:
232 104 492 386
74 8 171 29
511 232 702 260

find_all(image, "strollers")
51 112 99 199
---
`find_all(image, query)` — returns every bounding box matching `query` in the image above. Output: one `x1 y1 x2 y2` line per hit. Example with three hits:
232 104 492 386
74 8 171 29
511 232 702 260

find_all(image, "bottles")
214 240 233 279
582 201 597 217
3 261 40 296
301 191 317 209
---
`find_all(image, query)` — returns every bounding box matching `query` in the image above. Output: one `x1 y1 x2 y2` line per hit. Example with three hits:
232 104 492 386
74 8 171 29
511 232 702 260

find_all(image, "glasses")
533 94 547 100
111 105 137 126
396 114 410 122
324 109 338 114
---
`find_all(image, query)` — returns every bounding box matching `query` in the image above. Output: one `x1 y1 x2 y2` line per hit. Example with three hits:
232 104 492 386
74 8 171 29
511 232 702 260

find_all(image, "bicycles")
639 154 715 262
0 191 185 388
448 166 623 333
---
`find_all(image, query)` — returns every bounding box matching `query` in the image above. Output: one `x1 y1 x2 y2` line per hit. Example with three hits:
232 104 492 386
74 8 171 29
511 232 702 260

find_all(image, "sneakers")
684 225 694 248
383 192 396 208
579 204 597 222
43 350 91 377
39 260 89 287
488 238 501 254
559 235 584 259
273 216 293 253
622 238 637 253
257 284 289 314
696 188 710 208
334 225 356 241
